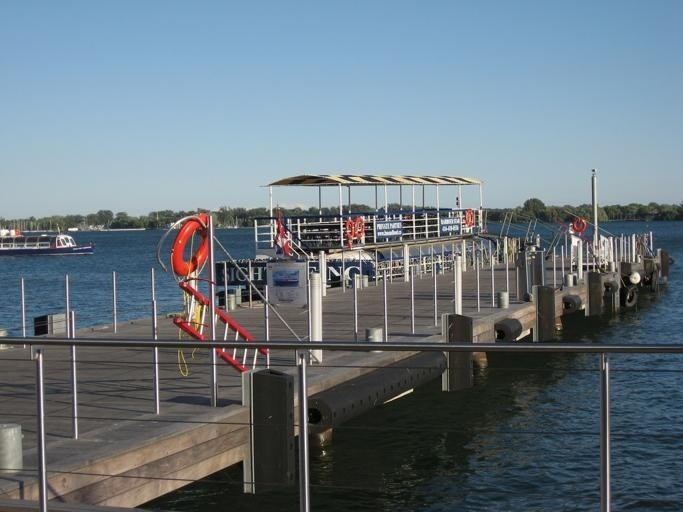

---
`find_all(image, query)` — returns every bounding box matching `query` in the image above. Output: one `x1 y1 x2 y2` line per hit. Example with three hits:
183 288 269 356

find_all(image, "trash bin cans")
608 262 615 272
636 254 641 263
565 273 574 287
0 424 23 474
365 327 384 353
498 291 509 308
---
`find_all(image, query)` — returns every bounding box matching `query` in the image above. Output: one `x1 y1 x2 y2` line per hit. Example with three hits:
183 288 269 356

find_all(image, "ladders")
173 282 269 372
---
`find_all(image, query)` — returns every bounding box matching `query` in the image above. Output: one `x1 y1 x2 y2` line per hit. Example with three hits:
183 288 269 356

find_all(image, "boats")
215 170 544 309
0 234 96 257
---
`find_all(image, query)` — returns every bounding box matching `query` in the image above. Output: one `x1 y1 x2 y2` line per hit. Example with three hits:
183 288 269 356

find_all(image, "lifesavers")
573 217 586 233
345 220 356 238
464 209 475 226
355 217 365 240
171 214 210 277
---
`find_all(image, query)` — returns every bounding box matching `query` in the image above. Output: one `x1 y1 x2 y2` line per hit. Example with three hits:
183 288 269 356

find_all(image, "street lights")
591 168 598 248
